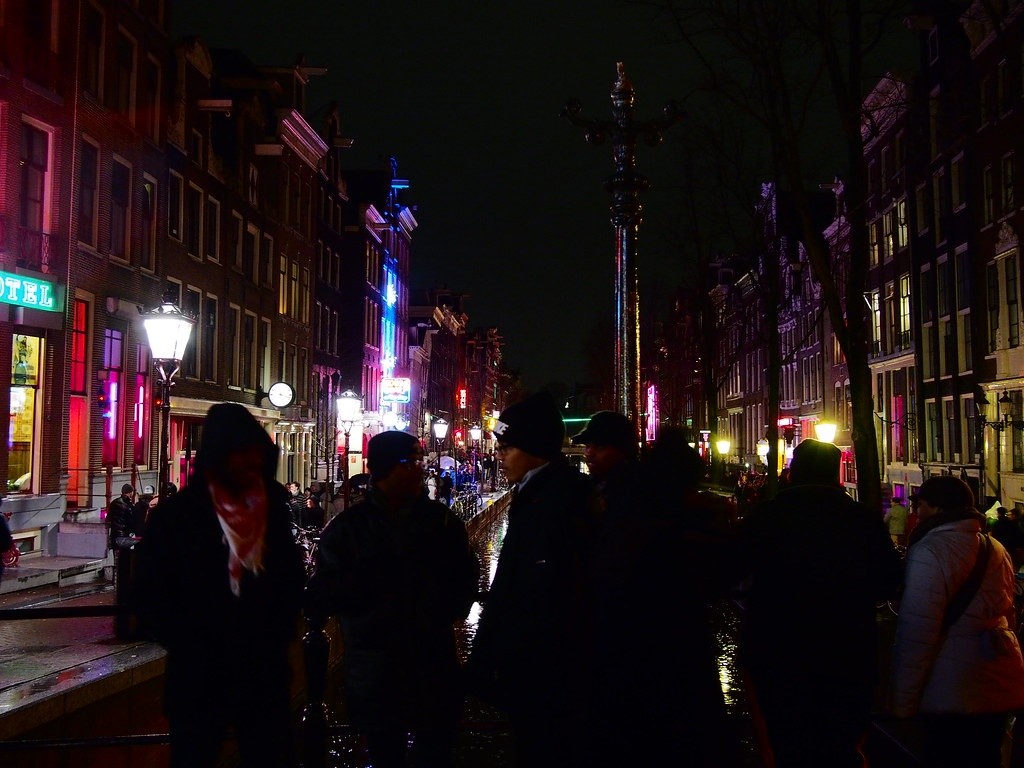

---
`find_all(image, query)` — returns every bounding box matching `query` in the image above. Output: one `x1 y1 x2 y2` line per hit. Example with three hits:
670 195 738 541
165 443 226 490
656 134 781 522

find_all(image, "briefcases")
869 713 934 768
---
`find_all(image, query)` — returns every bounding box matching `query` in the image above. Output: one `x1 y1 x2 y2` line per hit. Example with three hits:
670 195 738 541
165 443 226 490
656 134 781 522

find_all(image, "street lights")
137 287 198 505
469 424 482 492
432 418 451 501
335 388 362 512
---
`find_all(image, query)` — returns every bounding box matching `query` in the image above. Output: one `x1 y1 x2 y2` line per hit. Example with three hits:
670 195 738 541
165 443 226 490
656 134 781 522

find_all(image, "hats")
493 403 565 460
144 485 153 494
122 483 133 494
366 430 421 470
908 476 974 509
571 411 634 446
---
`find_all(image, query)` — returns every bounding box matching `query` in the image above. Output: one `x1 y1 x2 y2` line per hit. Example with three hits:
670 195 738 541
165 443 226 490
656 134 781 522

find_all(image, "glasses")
494 444 512 452
395 457 425 465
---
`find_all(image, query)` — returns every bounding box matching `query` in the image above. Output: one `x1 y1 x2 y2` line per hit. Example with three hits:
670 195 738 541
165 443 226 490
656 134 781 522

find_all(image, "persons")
883 475 1023 767
452 392 611 768
112 402 338 767
0 401 1024 633
298 431 481 768
569 410 727 767
727 436 909 768
